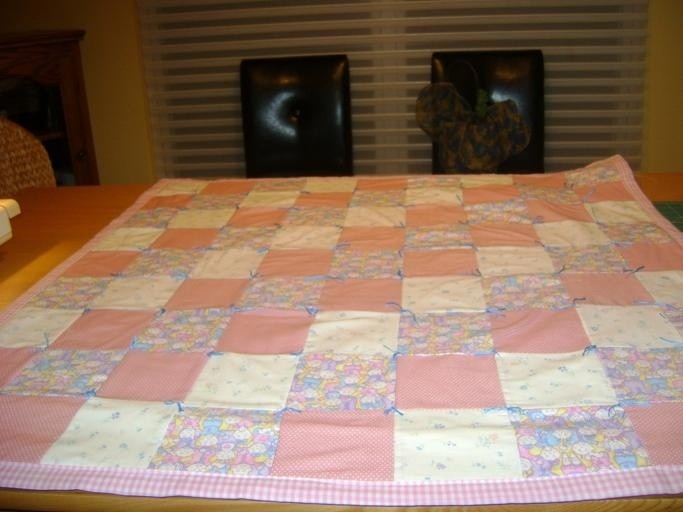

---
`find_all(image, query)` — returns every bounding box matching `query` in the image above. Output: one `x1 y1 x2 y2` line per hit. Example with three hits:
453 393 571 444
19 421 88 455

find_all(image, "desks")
1 171 682 512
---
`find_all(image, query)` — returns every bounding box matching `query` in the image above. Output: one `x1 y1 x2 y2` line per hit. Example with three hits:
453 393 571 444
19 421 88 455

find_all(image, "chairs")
431 50 544 174
239 55 354 177
